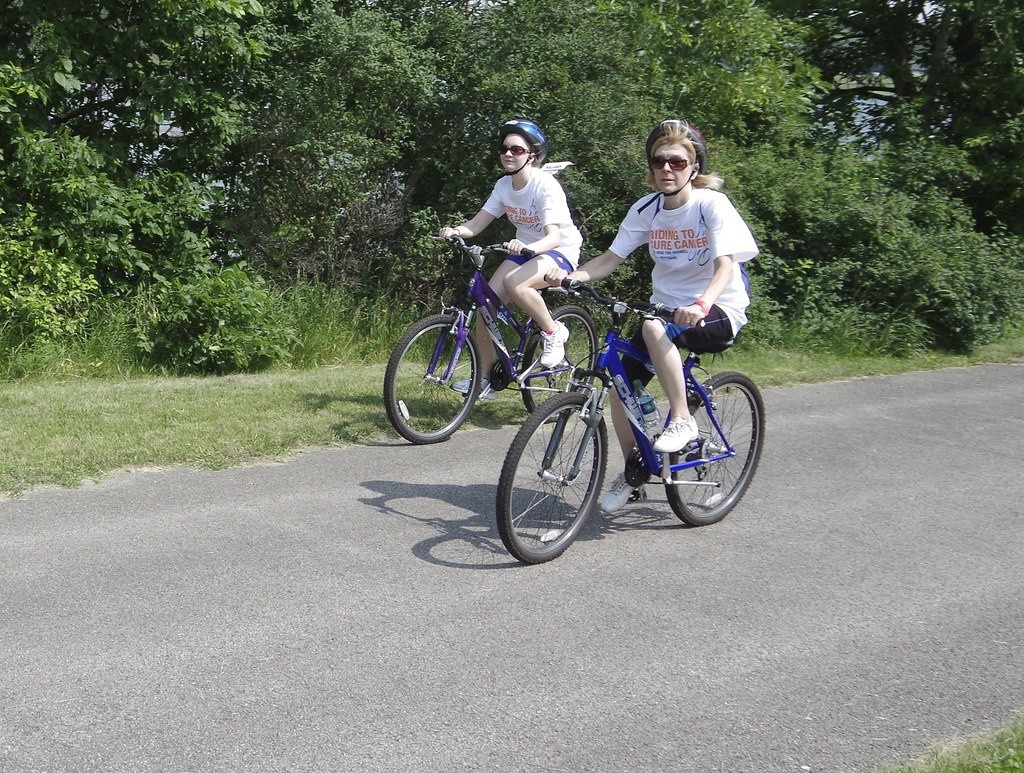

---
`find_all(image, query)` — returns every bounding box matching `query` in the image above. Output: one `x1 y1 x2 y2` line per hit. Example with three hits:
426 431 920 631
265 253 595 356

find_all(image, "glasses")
649 157 691 171
497 144 529 155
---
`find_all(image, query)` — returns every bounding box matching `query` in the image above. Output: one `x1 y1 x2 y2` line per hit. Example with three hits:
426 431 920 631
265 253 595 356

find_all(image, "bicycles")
495 275 765 565
383 235 600 444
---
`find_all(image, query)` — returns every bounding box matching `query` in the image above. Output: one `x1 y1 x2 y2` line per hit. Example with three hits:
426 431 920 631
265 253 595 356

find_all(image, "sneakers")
652 416 698 453
600 472 635 513
539 321 569 368
448 377 496 400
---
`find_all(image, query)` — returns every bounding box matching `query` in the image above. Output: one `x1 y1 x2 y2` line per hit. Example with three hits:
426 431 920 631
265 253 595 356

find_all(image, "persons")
439 119 583 400
545 120 759 513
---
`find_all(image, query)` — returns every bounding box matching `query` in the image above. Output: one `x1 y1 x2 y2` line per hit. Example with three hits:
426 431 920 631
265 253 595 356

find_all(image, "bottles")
632 379 660 428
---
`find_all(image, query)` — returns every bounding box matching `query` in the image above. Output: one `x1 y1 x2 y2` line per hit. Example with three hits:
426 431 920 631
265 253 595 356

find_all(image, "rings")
686 320 690 323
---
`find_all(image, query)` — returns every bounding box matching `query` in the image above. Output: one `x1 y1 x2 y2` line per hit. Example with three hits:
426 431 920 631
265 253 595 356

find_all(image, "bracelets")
694 300 710 316
453 228 461 236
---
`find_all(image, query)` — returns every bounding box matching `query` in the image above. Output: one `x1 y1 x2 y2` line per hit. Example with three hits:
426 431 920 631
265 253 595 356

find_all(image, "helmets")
499 119 547 162
646 119 706 174
